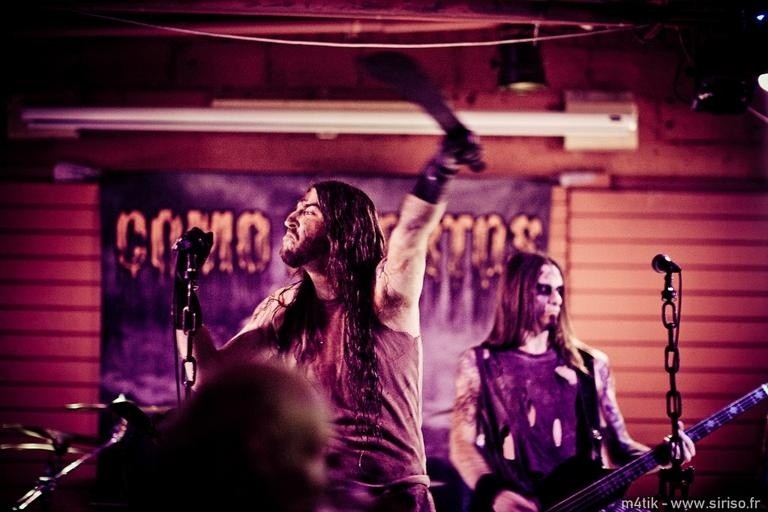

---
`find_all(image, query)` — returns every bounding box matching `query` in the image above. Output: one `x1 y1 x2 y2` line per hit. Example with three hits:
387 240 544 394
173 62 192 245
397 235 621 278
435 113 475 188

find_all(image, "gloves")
403 123 485 210
170 227 218 332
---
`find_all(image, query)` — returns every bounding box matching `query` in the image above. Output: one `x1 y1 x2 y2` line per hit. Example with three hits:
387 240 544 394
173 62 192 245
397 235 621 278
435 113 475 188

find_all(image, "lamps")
497 28 546 92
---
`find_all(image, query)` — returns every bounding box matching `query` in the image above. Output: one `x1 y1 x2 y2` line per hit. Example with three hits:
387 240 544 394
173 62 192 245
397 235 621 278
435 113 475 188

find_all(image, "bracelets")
471 473 508 505
405 156 462 209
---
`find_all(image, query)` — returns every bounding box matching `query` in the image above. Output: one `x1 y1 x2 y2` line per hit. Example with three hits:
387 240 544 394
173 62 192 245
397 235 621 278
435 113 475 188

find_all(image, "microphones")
651 254 682 275
182 238 201 283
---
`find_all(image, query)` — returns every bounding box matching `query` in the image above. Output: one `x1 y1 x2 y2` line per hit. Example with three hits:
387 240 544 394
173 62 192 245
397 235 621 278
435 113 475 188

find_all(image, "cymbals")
2 425 101 444
64 403 172 415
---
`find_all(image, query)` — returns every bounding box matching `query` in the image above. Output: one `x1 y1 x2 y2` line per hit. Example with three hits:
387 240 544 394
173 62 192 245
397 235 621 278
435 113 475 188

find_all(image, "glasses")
536 283 565 298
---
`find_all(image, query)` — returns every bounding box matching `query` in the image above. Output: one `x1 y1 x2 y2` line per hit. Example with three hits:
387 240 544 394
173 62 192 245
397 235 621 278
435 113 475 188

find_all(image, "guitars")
466 384 768 512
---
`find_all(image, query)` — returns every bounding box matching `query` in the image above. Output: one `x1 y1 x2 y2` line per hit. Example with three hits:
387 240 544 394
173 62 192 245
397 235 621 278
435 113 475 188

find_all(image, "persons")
450 246 697 511
165 124 490 512
129 358 347 510
